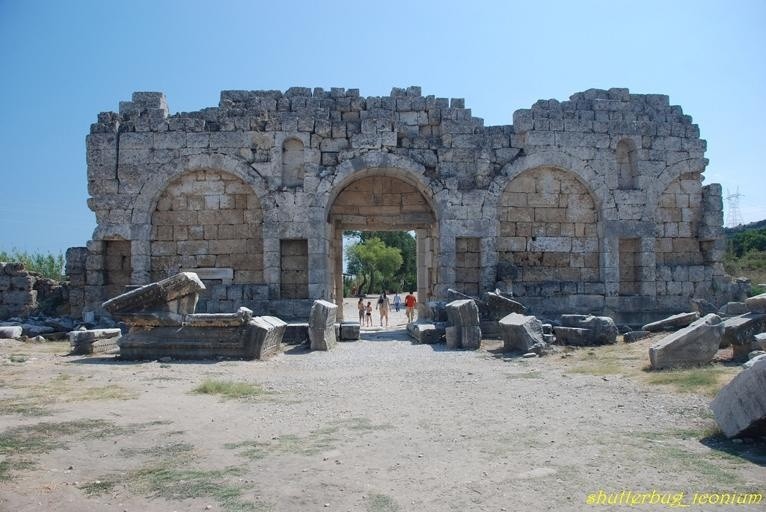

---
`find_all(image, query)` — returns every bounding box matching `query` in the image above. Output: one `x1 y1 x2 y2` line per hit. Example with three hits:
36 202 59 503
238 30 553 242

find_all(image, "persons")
357 297 366 326
393 292 401 312
365 302 372 327
404 291 416 323
375 293 390 326
